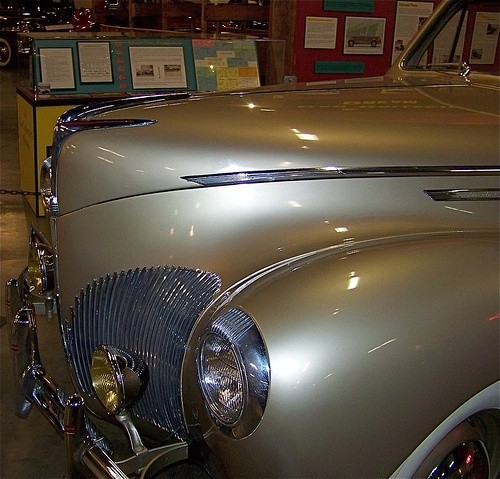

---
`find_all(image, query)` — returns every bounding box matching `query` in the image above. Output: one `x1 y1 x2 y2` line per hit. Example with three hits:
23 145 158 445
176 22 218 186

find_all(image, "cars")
0 0 500 479
0 0 96 70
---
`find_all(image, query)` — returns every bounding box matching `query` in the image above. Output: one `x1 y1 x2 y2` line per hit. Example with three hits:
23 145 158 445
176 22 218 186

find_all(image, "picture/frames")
344 15 387 55
37 46 75 91
128 46 186 90
75 42 114 84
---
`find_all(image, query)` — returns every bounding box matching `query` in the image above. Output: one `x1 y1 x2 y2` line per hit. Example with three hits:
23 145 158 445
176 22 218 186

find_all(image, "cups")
37 82 50 98
